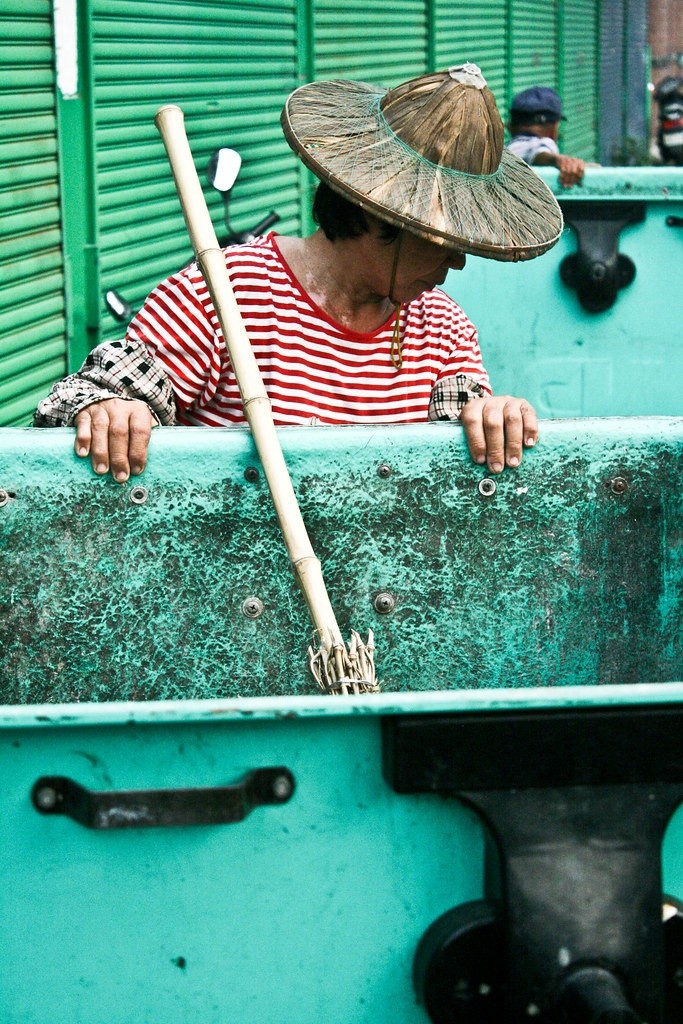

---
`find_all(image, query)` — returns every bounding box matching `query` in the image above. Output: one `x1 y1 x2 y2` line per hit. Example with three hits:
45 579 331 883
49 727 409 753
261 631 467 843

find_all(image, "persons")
506 87 602 187
32 62 538 484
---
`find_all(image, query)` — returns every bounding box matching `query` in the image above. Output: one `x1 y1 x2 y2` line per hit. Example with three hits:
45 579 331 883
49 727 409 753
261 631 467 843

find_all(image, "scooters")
104 148 281 324
652 53 683 168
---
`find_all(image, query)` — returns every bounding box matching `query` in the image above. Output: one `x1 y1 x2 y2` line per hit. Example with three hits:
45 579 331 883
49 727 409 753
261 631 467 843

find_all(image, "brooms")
153 103 385 696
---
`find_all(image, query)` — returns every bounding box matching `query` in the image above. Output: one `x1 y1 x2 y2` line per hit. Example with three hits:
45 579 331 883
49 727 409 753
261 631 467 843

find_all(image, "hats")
509 87 568 126
279 62 564 262
